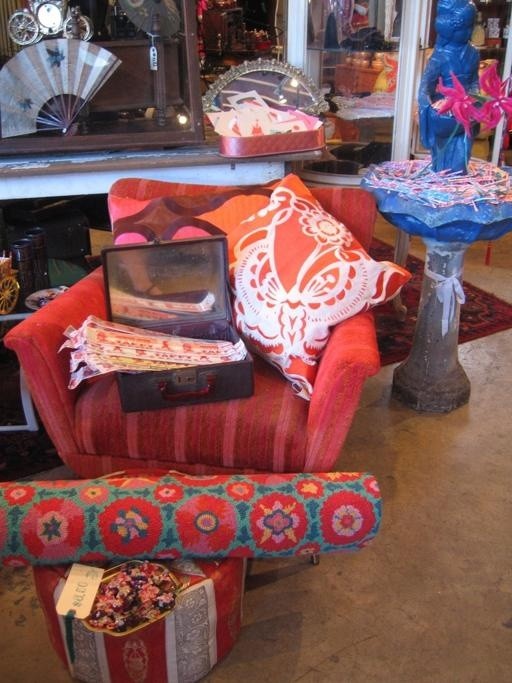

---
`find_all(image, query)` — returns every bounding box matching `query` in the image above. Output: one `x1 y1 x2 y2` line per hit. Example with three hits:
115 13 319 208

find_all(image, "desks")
362 158 512 414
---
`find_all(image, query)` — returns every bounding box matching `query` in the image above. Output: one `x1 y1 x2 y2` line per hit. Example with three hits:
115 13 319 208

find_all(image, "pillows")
220 171 412 401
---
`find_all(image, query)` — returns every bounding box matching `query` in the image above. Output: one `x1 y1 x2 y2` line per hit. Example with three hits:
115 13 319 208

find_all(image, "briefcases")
100 233 258 413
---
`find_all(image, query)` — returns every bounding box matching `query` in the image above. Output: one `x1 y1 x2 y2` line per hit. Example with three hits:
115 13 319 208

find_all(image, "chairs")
4 177 380 478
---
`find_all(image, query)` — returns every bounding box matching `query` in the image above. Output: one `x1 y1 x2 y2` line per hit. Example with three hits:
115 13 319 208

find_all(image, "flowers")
415 63 512 179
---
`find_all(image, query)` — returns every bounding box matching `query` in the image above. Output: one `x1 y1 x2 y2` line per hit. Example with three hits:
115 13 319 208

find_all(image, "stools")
28 469 248 681
